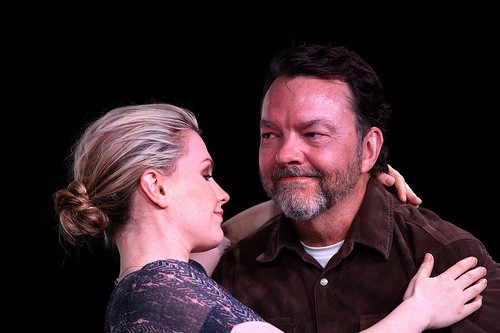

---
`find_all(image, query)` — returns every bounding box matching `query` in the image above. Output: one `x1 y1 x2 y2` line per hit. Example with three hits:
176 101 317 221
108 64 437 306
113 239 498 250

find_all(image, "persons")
52 103 489 333
206 41 500 333
